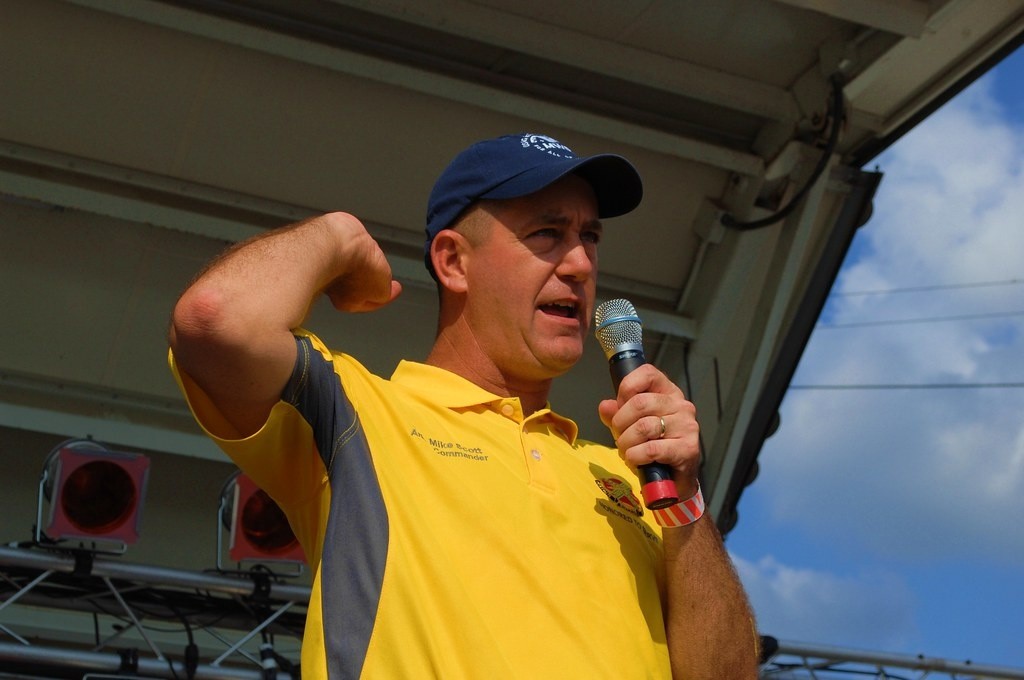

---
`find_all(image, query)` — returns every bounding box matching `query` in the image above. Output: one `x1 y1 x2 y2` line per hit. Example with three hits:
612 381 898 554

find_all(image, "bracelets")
652 477 705 528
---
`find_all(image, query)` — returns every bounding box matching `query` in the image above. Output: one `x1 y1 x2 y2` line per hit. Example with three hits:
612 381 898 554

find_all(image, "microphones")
594 299 679 511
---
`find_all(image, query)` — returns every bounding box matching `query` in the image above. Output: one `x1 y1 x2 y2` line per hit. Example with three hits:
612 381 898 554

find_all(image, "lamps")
41 440 149 554
222 468 308 563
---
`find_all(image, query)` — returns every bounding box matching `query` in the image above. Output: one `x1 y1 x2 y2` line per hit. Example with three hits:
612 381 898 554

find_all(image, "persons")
168 133 759 679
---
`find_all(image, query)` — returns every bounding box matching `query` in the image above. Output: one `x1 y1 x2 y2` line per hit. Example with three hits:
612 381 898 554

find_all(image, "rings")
658 415 666 439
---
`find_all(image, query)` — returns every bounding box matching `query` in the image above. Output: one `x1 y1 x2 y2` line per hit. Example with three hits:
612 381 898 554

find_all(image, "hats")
423 133 644 283
